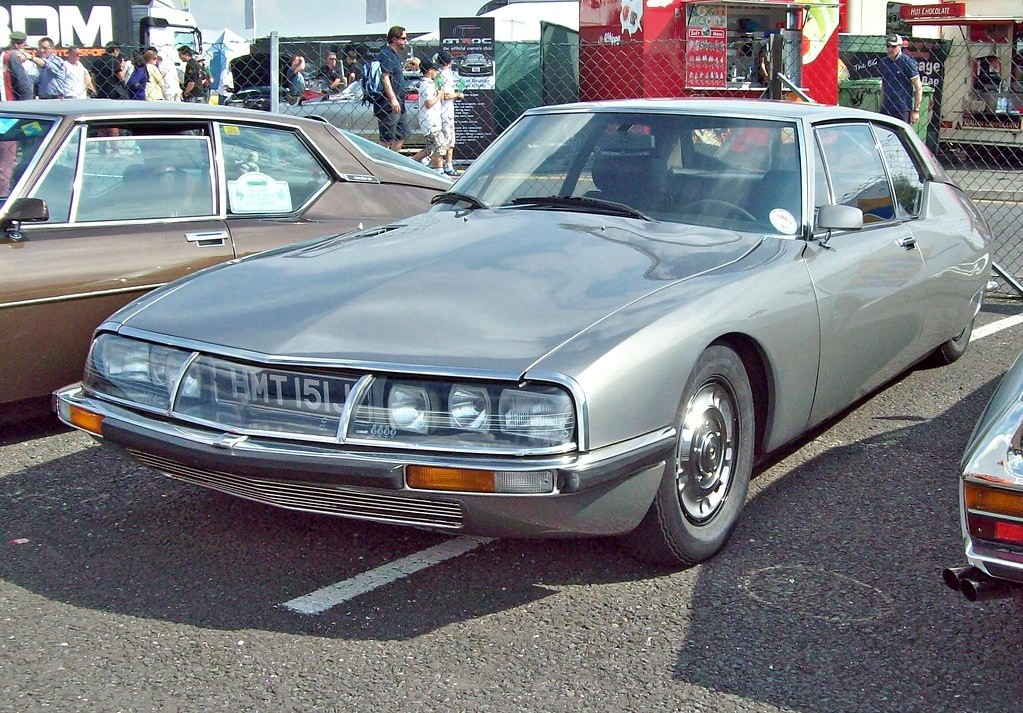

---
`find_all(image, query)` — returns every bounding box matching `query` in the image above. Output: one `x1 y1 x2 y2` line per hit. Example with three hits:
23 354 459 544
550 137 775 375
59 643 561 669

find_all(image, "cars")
451 55 464 71
280 70 429 150
942 349 1022 606
53 100 992 576
1 98 463 431
223 51 304 116
459 54 493 77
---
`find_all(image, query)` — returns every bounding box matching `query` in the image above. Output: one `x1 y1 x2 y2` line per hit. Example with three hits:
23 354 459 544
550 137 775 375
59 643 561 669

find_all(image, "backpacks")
361 49 389 106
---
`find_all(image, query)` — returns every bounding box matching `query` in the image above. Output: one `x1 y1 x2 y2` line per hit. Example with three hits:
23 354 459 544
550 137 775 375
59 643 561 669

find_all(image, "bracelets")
29 55 33 61
913 109 920 112
456 92 460 99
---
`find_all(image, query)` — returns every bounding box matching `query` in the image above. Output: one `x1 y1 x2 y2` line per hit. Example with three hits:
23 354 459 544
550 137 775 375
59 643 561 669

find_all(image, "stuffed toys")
229 151 259 180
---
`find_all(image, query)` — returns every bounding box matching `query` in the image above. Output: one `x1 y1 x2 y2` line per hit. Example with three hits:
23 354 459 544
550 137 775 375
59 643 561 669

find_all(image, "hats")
419 58 436 72
438 52 454 64
347 51 357 59
9 31 26 41
886 34 902 45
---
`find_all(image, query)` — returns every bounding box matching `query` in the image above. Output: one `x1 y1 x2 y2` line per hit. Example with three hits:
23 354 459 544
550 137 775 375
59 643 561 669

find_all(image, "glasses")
328 58 336 60
396 36 409 41
150 58 157 60
887 44 897 48
10 41 25 44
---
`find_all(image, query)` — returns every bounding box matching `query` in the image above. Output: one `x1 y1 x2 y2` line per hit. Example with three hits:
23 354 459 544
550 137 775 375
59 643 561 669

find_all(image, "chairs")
582 146 671 214
744 169 800 225
102 165 177 218
16 164 75 223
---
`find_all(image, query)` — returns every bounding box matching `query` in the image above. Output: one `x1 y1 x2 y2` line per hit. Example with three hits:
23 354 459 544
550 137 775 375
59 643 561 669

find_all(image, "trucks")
0 0 203 103
579 0 1022 168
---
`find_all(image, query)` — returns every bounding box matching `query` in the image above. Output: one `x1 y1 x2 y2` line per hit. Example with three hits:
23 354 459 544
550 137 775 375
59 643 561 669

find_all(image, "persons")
287 54 306 105
0 48 34 208
434 51 464 176
315 52 341 102
413 58 447 176
405 57 422 70
878 34 922 125
372 25 413 152
758 31 779 86
177 45 214 104
9 31 182 160
346 51 362 85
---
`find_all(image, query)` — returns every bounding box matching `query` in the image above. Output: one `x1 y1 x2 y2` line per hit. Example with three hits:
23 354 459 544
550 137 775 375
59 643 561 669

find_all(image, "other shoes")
438 171 450 179
444 169 461 179
